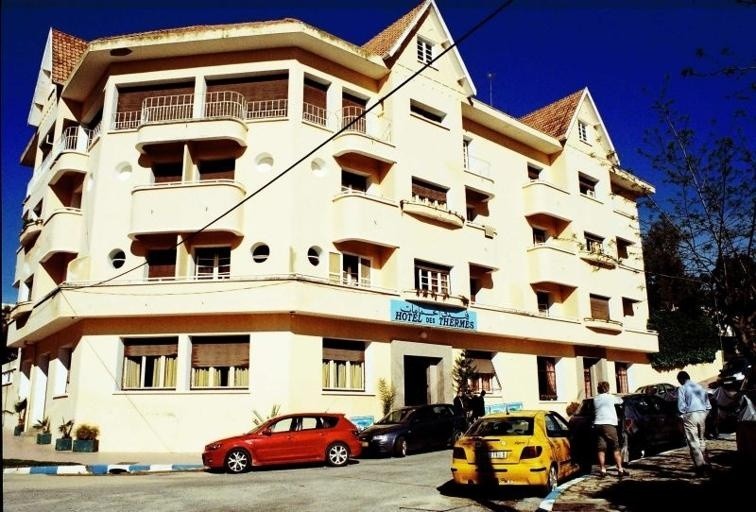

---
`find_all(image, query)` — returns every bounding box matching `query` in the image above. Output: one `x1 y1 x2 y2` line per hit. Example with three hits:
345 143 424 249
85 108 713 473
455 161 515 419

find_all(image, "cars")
359 402 466 457
199 410 363 473
451 379 729 495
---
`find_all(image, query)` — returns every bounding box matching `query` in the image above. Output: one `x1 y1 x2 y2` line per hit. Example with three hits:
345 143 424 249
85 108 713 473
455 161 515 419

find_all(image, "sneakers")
601 471 608 478
617 470 630 477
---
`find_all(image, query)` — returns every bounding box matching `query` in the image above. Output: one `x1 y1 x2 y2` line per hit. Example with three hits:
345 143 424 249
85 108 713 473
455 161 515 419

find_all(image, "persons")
677 370 713 474
453 389 468 435
471 387 486 419
593 381 629 477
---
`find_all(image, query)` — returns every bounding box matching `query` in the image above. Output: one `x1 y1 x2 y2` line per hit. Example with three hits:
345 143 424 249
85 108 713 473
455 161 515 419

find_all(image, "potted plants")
13 402 25 436
33 417 99 453
